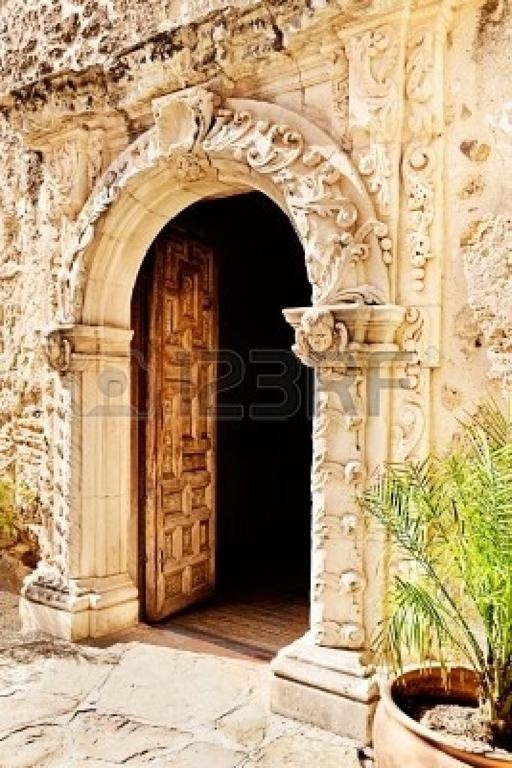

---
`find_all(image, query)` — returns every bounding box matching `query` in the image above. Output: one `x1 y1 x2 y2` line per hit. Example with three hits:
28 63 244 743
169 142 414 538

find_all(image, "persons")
294 306 348 357
36 327 62 364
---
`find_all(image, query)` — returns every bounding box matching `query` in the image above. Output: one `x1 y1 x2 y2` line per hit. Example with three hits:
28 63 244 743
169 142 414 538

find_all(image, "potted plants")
354 399 512 768
